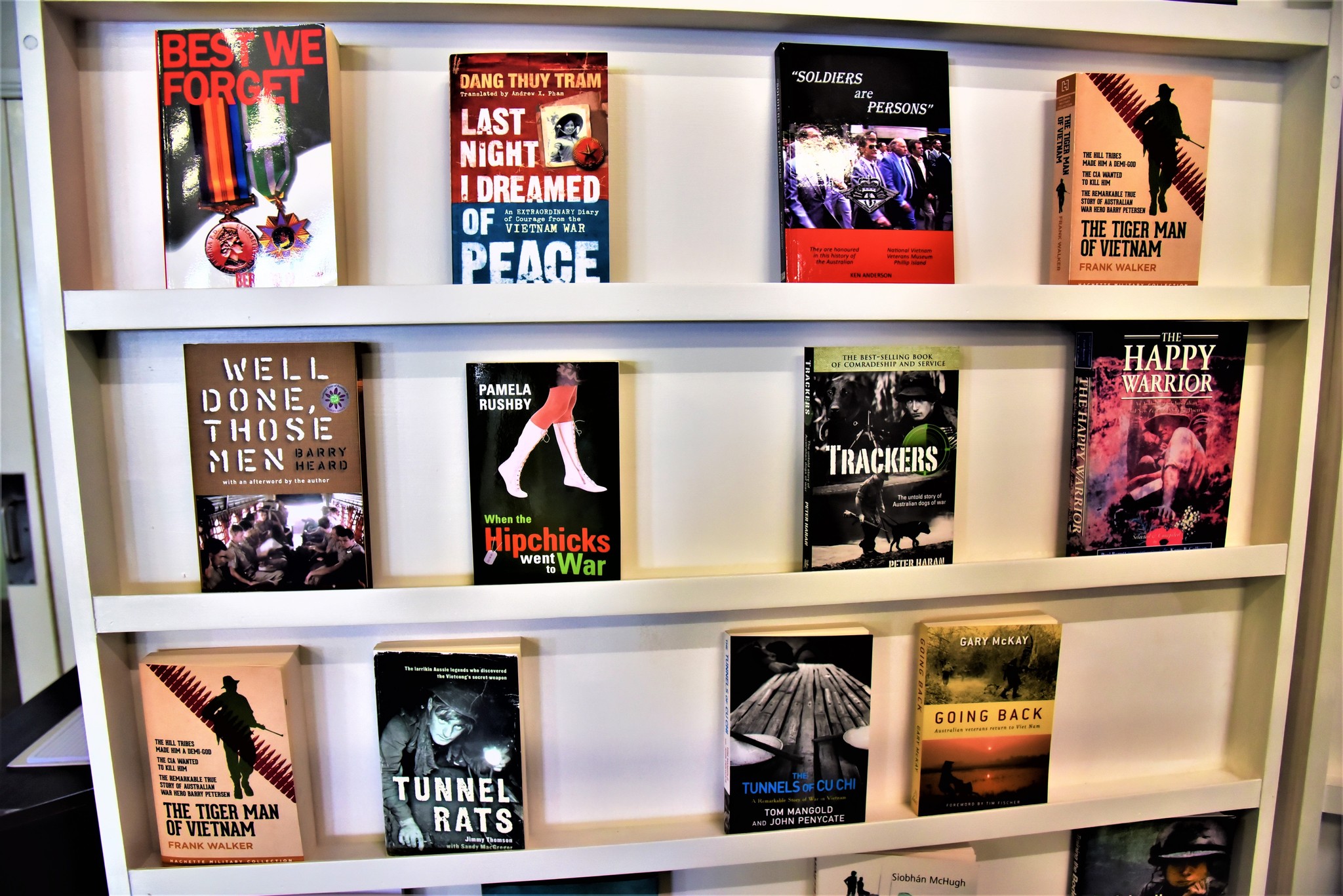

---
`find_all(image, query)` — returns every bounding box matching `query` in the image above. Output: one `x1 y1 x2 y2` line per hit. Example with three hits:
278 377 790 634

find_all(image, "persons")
200 499 368 590
1140 818 1230 895
855 370 957 557
551 113 582 162
1126 402 1209 519
783 123 953 231
803 346 962 572
381 689 510 850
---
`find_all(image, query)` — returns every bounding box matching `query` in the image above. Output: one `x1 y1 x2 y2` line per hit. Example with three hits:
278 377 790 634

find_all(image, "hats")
1155 83 1175 98
894 369 943 403
428 681 483 726
220 676 240 689
1144 402 1191 435
554 113 583 139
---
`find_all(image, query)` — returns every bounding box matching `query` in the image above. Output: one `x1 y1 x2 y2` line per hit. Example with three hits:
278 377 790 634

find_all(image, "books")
910 612 1062 818
138 645 318 865
774 42 955 285
481 872 673 896
1066 811 1237 896
154 22 339 288
450 53 610 285
724 626 873 836
813 840 977 896
1048 72 1213 287
183 342 372 593
1065 321 1248 558
466 361 621 584
373 636 527 858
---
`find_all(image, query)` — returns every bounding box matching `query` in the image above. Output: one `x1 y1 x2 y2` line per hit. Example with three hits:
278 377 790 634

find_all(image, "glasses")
868 137 878 143
866 144 880 150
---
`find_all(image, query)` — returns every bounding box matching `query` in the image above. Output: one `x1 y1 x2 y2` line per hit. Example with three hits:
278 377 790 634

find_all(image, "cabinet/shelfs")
12 0 1340 894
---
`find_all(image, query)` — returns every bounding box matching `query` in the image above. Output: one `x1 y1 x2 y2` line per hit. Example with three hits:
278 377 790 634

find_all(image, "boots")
494 419 551 500
553 420 609 496
